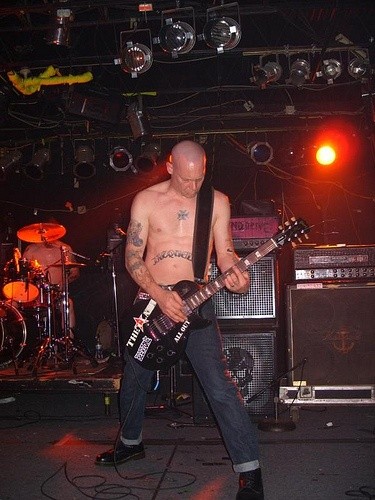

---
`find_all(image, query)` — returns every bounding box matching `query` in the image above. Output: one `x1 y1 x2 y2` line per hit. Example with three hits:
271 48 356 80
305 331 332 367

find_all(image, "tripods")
18 240 100 380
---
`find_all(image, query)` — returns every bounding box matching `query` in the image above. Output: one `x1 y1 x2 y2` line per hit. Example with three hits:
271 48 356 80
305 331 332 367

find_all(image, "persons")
95 140 264 500
22 218 79 339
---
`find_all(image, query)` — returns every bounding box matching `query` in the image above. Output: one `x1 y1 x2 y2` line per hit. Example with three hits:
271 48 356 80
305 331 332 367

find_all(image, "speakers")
207 252 280 328
285 282 375 400
191 328 279 423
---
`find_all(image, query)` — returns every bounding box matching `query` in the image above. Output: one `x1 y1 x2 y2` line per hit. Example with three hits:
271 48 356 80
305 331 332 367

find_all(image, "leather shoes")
235 469 264 500
95 442 146 465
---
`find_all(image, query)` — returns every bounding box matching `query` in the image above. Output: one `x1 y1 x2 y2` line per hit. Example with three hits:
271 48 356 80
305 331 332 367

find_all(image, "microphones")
303 356 308 364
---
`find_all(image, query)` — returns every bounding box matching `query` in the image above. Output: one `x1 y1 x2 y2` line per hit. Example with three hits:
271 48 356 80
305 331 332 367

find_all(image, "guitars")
121 216 312 374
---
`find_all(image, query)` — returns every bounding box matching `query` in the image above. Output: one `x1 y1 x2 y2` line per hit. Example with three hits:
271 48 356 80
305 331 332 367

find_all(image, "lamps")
250 49 368 89
118 0 242 78
45 10 75 50
0 135 96 182
110 101 161 172
247 134 273 165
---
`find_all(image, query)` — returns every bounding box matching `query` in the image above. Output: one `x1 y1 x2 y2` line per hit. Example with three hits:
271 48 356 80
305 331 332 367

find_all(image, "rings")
229 283 235 287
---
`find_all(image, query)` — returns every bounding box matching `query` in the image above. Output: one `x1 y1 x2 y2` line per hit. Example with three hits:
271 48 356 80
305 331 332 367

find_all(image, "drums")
21 283 61 311
0 301 27 366
2 257 42 303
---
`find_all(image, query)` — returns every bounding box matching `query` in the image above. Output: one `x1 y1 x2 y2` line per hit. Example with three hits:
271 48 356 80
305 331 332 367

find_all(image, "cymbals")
48 260 88 267
17 223 65 244
